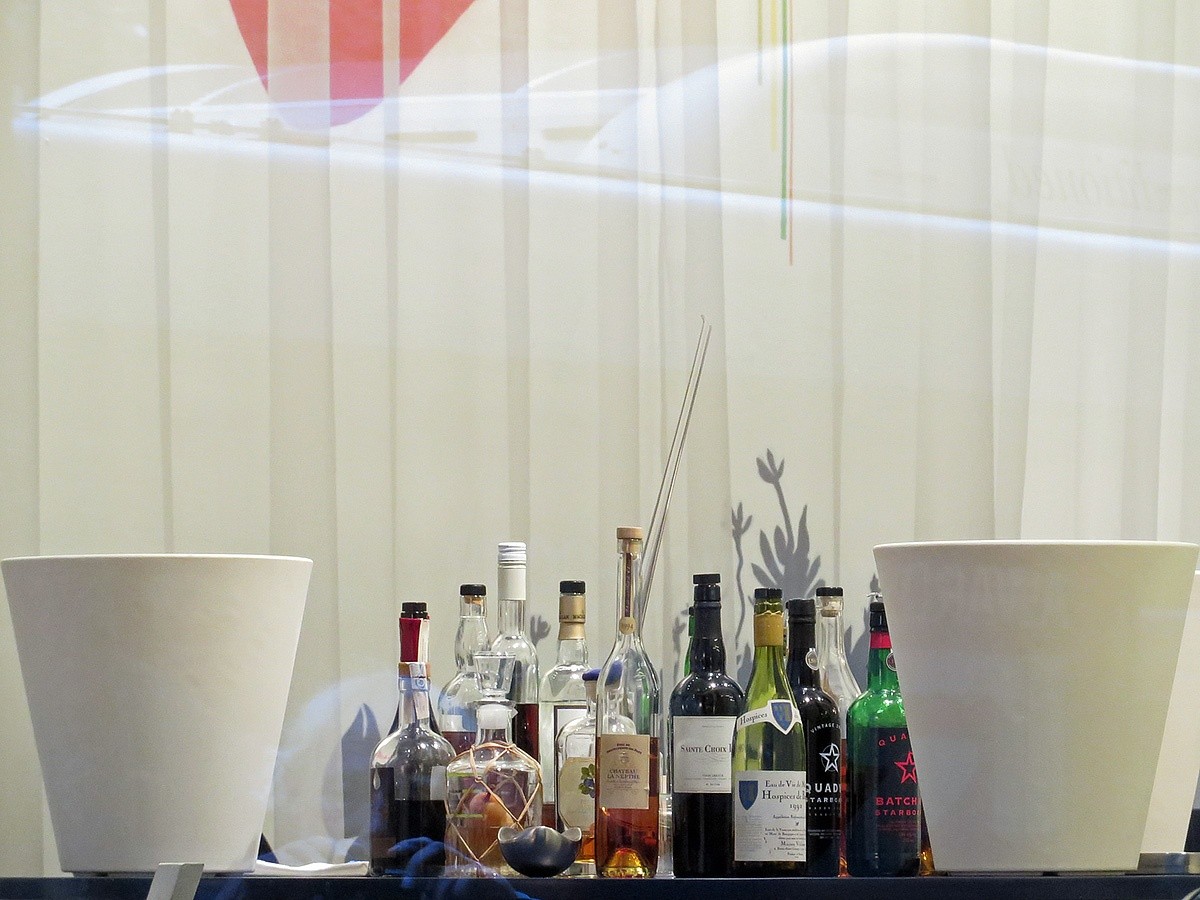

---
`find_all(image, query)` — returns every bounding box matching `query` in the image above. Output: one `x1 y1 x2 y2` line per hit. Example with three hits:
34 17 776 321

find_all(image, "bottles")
435 584 490 754
815 587 861 876
538 581 594 833
784 599 840 876
388 602 439 733
556 670 600 873
596 527 661 879
847 603 921 876
368 662 456 877
489 542 538 760
731 588 807 876
667 575 746 877
445 652 543 877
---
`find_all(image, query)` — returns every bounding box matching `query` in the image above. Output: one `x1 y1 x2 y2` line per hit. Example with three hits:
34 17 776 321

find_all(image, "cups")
497 825 582 876
1 554 314 870
873 540 1199 871
